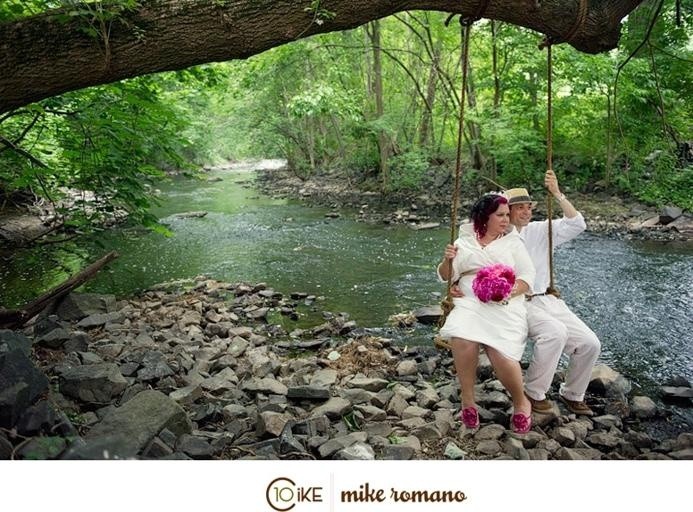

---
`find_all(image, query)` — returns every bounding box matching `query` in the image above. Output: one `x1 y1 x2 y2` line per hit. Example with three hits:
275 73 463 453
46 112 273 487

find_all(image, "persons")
437 195 536 434
451 170 601 416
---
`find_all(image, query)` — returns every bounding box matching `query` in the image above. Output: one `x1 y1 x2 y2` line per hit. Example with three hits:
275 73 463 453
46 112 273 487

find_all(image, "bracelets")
555 193 565 204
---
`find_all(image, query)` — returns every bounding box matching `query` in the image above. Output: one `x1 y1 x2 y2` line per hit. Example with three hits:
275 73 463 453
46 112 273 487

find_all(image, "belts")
524 292 551 297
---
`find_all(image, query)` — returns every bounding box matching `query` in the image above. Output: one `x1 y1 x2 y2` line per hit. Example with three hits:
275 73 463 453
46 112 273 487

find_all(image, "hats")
503 188 539 205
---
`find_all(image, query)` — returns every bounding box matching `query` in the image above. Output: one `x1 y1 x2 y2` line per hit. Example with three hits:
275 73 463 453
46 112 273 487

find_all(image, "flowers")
470 262 517 307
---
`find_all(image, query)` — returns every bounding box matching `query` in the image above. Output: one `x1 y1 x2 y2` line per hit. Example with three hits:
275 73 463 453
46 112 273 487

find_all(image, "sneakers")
559 392 594 415
510 402 532 433
524 390 554 413
461 406 480 431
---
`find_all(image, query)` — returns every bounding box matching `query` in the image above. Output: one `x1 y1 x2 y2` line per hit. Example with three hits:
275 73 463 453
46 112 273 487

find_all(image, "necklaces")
476 233 504 249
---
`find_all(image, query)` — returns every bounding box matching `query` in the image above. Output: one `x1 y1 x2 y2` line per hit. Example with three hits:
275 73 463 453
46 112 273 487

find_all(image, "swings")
433 21 562 353
